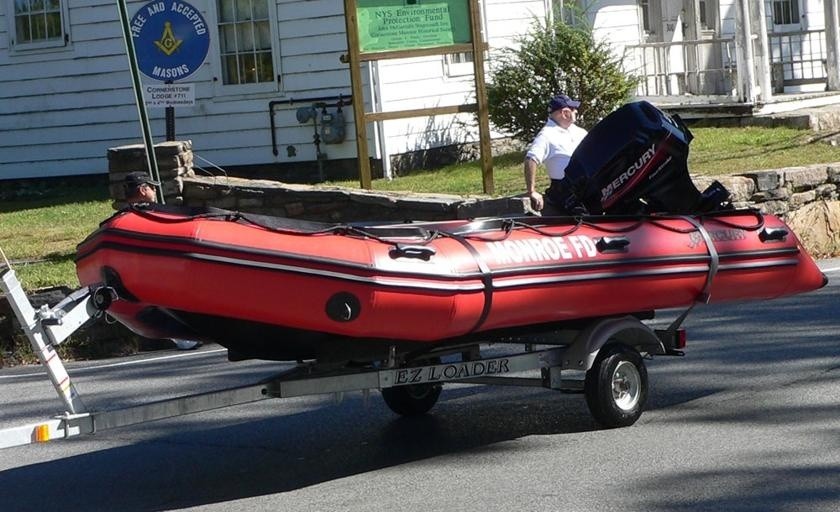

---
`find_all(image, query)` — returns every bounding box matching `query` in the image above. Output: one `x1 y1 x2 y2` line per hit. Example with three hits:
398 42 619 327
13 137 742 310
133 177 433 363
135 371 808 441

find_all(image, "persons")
524 96 589 210
123 172 160 206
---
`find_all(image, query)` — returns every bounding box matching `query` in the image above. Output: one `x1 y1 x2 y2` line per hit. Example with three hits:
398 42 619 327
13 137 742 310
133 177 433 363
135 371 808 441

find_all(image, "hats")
546 94 581 115
123 171 159 190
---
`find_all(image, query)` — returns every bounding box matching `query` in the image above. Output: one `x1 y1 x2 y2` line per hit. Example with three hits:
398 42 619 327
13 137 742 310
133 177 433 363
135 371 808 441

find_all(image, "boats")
69 101 829 352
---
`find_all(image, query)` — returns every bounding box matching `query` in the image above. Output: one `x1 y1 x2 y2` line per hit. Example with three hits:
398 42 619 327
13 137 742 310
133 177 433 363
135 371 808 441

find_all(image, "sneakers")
168 338 198 349
